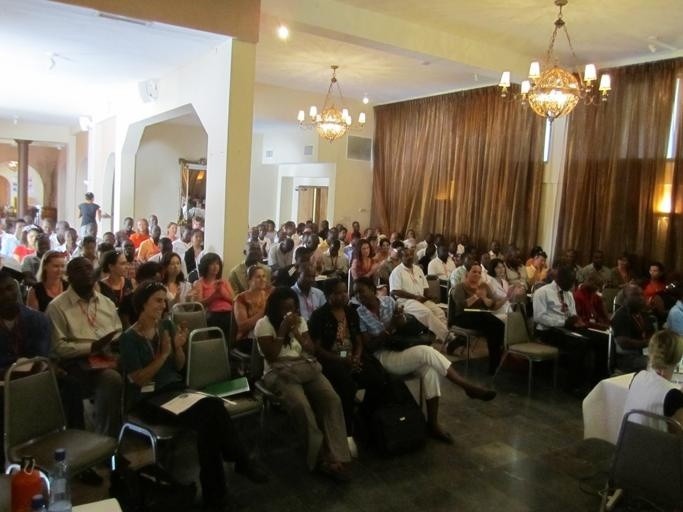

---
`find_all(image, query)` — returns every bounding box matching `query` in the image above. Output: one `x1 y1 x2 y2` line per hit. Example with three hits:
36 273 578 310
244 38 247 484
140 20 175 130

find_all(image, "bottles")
30 492 47 511
11 455 51 511
47 447 73 512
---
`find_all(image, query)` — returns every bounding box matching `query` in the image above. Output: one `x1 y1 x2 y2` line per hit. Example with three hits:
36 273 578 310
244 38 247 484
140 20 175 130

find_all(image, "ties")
559 290 567 312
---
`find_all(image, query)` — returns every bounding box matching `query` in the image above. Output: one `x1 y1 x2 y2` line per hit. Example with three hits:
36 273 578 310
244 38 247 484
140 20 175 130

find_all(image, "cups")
0 472 11 511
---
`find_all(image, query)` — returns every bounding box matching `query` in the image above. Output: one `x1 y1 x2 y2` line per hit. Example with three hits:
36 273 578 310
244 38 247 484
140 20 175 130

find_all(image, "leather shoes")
465 388 495 401
234 463 271 485
424 422 453 444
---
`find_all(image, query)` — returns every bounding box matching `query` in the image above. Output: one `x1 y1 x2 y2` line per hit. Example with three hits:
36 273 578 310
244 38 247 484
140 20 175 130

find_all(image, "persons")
78 193 102 240
616 329 683 433
182 199 206 222
483 241 683 400
1 214 497 512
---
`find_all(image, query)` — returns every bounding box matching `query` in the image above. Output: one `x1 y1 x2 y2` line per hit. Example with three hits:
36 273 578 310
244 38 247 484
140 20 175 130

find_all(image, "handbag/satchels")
384 328 409 351
273 356 323 384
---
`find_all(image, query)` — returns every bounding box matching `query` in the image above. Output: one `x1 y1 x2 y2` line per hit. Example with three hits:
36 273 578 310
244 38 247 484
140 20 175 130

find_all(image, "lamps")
298 66 366 143
499 0 612 125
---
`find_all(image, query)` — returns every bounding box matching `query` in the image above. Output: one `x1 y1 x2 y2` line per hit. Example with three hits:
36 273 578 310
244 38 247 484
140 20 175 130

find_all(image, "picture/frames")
179 159 207 219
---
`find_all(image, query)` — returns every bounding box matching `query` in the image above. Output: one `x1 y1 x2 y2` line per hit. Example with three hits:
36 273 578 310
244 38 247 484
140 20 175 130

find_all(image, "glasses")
145 282 164 289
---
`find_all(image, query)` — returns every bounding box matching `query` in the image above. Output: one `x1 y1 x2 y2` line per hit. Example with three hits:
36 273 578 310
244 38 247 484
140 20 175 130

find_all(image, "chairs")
0 268 683 511
